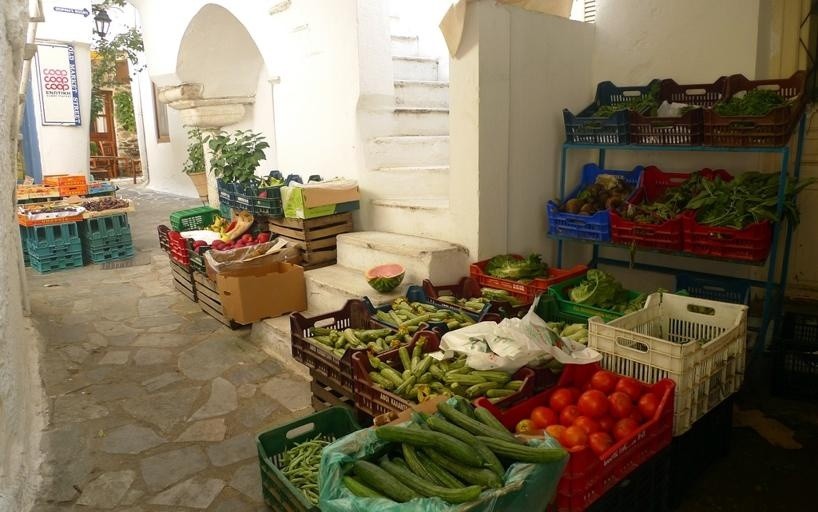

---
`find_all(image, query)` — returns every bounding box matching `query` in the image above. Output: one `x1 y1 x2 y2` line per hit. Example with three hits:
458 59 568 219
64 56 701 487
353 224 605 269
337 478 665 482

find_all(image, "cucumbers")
310 287 588 502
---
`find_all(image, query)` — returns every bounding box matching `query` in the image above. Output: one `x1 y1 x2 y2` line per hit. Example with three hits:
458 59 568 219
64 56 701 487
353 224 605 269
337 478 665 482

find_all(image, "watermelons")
365 263 406 293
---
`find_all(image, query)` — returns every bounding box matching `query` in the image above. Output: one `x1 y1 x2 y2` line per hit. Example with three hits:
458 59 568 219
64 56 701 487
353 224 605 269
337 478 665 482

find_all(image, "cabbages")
568 269 622 306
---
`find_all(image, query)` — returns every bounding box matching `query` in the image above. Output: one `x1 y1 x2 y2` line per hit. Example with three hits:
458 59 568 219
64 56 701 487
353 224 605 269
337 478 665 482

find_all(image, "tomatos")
516 370 660 455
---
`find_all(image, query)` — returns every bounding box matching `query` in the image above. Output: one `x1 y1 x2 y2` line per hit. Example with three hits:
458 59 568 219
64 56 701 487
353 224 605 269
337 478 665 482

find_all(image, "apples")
193 233 268 254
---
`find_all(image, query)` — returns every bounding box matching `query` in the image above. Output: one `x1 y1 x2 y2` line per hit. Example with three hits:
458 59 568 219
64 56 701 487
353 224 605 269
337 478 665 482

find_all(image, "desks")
90 156 115 179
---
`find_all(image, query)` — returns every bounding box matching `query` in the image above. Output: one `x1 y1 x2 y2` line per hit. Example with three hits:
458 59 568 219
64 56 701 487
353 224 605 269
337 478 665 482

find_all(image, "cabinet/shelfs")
559 113 807 348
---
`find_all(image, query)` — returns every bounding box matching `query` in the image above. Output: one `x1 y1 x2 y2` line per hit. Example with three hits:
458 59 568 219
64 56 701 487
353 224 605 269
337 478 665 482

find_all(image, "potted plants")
182 128 208 201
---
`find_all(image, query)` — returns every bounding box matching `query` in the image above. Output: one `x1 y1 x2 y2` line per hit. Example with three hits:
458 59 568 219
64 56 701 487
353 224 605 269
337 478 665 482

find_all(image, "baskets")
423 277 534 321
351 330 533 418
547 273 643 322
255 252 751 512
255 406 367 512
475 363 675 512
289 299 430 400
170 206 221 232
563 69 808 147
767 312 817 401
468 252 586 304
364 284 490 345
546 163 773 262
587 292 749 437
499 292 616 327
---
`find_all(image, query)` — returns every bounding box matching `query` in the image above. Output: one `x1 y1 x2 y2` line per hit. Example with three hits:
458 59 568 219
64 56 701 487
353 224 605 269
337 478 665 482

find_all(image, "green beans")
282 432 330 504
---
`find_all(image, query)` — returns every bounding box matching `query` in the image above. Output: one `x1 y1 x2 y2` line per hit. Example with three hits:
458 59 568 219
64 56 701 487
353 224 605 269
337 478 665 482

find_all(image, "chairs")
99 139 129 178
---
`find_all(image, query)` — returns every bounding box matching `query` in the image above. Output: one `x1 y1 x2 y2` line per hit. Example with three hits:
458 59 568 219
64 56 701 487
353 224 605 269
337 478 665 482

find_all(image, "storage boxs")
216 260 309 326
280 179 360 220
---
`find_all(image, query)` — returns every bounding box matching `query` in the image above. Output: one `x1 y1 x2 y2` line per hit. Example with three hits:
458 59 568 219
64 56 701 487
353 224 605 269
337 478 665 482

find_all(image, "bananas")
211 215 232 241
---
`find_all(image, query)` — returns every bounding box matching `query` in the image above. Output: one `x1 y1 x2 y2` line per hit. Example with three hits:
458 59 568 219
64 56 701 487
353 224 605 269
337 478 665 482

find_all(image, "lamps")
93 8 112 38
